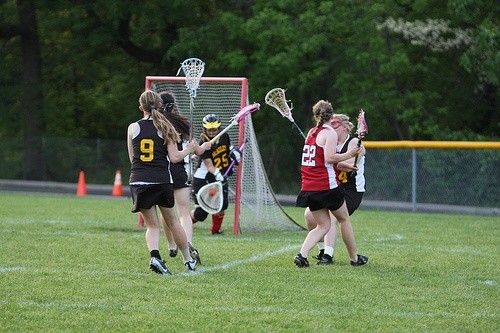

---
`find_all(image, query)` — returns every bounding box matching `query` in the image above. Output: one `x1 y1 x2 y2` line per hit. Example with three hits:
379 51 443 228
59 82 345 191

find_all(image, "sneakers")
184 259 197 271
150 256 171 275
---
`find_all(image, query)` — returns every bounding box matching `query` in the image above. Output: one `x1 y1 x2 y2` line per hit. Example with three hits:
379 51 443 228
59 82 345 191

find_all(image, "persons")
127 90 197 275
304 114 366 265
191 114 242 235
294 100 368 267
159 92 210 262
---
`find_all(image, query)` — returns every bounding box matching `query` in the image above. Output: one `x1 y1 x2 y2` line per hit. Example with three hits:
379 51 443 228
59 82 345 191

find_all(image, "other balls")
210 189 215 195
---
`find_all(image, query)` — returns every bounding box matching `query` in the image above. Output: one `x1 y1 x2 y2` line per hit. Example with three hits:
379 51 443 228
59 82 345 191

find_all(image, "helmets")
202 113 222 128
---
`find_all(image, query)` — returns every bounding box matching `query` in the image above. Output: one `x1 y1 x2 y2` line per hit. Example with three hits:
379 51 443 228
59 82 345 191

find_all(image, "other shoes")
350 253 368 266
169 246 178 256
316 248 325 260
316 254 333 264
210 229 225 234
188 242 201 264
294 252 308 267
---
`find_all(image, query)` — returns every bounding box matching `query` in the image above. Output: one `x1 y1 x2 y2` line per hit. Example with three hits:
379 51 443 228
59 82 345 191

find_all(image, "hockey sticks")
350 108 367 177
179 57 206 186
195 137 250 215
190 103 260 162
263 87 306 139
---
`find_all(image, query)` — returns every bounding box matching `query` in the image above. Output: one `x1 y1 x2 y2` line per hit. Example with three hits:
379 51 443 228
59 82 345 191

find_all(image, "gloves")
214 167 227 185
230 146 243 165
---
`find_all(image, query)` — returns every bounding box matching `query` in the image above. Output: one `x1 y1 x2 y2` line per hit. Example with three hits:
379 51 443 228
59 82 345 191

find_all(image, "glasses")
330 119 350 129
